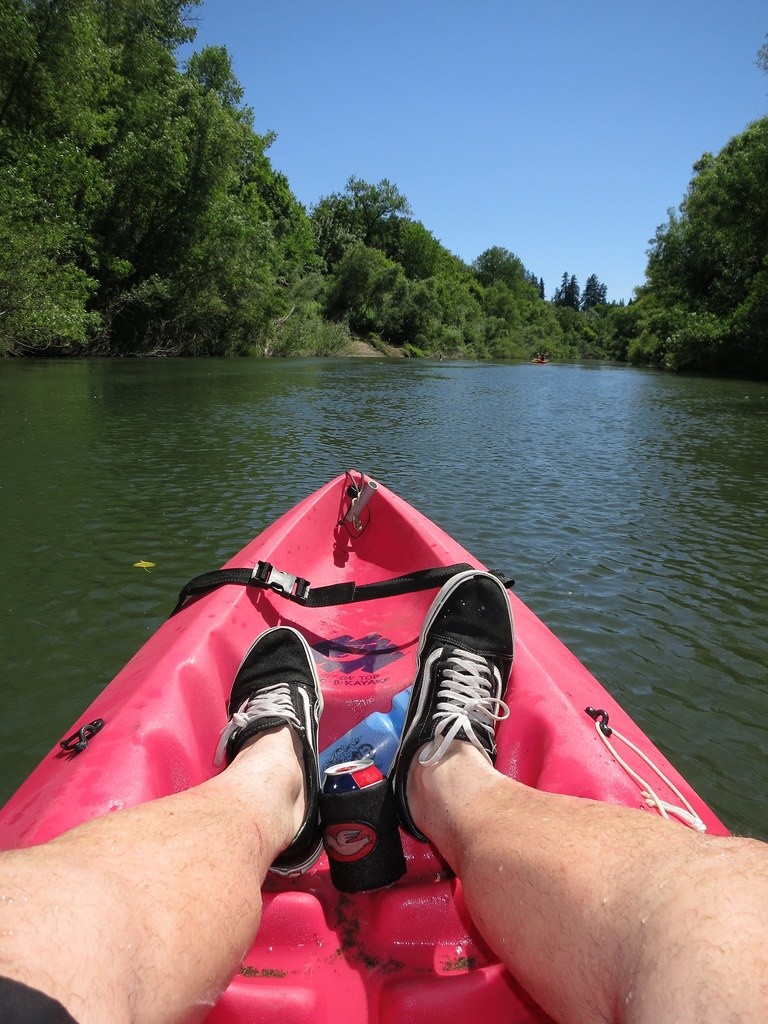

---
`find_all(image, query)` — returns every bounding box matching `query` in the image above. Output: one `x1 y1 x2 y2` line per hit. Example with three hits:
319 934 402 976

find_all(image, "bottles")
319 686 414 784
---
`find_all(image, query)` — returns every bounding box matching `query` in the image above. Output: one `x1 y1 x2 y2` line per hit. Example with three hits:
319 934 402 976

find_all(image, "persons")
0 569 768 1024
539 352 546 360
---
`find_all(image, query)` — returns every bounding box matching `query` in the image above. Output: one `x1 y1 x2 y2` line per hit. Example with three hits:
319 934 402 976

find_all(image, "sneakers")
213 626 324 878
386 570 515 842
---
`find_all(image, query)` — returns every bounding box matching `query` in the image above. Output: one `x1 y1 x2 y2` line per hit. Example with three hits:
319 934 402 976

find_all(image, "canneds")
321 759 384 794
